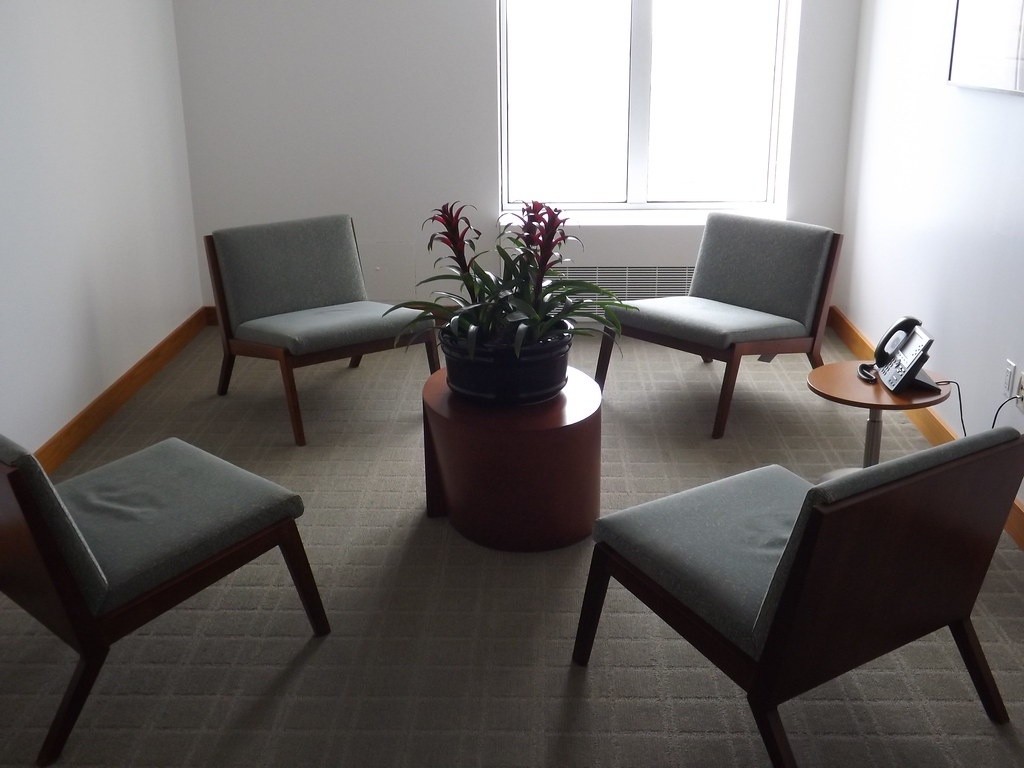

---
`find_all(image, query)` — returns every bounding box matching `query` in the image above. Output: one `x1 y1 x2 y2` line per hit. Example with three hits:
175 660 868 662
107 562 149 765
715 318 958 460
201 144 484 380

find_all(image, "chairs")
202 214 440 446
570 425 1024 768
0 436 331 768
593 212 844 438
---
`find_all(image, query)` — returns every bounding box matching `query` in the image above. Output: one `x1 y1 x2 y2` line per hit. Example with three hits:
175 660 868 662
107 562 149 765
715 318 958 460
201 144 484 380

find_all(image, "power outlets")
1015 371 1024 412
1002 359 1016 398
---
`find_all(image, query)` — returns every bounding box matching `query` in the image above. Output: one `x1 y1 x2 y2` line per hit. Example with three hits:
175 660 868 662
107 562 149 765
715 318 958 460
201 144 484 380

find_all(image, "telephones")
873 316 934 394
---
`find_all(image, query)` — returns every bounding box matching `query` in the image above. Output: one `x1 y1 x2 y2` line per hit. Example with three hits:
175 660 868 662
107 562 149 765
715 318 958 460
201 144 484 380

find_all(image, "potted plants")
381 198 640 391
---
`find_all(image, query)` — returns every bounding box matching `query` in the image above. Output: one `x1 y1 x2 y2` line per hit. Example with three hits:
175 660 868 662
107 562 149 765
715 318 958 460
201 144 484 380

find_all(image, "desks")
807 360 951 481
422 367 602 551
